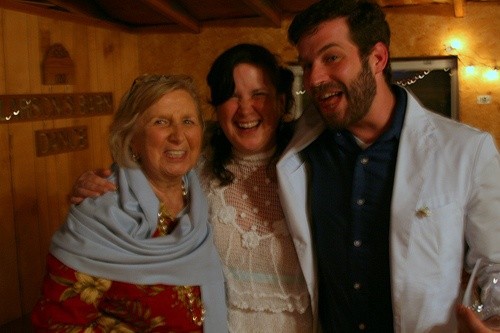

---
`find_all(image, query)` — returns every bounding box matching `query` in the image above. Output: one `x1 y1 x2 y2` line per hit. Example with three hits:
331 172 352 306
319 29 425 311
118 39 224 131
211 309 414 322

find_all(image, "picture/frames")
285 55 459 121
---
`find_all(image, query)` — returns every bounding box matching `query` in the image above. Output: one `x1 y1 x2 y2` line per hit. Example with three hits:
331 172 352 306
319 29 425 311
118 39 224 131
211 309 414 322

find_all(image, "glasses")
127 75 194 98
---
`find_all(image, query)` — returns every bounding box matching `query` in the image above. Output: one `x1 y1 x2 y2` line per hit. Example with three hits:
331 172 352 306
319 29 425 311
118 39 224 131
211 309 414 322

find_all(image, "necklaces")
158 180 206 326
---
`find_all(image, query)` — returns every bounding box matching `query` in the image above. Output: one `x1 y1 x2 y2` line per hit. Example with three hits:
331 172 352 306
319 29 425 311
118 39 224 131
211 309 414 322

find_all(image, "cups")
460 256 500 333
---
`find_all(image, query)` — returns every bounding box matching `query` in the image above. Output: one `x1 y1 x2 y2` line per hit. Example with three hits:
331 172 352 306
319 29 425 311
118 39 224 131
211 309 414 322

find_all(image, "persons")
275 0 500 333
65 41 315 333
31 72 228 333
